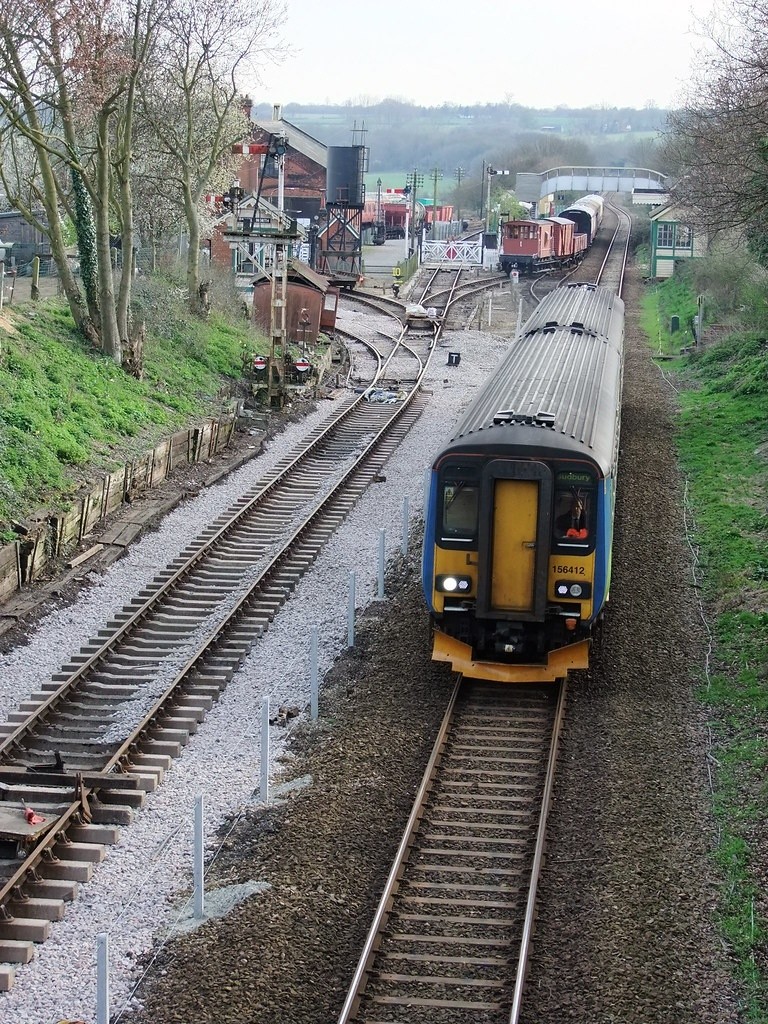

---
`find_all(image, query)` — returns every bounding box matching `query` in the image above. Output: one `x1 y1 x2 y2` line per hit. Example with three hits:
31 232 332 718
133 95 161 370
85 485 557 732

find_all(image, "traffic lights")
224 196 239 204
269 145 286 155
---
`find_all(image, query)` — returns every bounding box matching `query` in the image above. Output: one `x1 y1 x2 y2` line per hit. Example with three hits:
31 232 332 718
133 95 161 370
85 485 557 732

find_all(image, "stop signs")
296 358 309 372
253 356 267 370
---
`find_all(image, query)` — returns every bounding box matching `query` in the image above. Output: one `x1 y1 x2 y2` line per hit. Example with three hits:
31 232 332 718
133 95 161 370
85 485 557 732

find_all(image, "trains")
422 281 626 682
499 193 604 276
381 202 454 239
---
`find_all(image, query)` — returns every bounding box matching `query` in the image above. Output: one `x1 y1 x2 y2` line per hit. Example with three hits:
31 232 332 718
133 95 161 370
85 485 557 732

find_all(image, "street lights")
376 177 382 221
486 162 509 231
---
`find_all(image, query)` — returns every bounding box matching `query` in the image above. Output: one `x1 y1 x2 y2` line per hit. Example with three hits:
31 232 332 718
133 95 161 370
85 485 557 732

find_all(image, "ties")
574 518 578 530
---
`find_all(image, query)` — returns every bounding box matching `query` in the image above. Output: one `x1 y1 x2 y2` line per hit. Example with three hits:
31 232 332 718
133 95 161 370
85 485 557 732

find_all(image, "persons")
556 499 587 532
